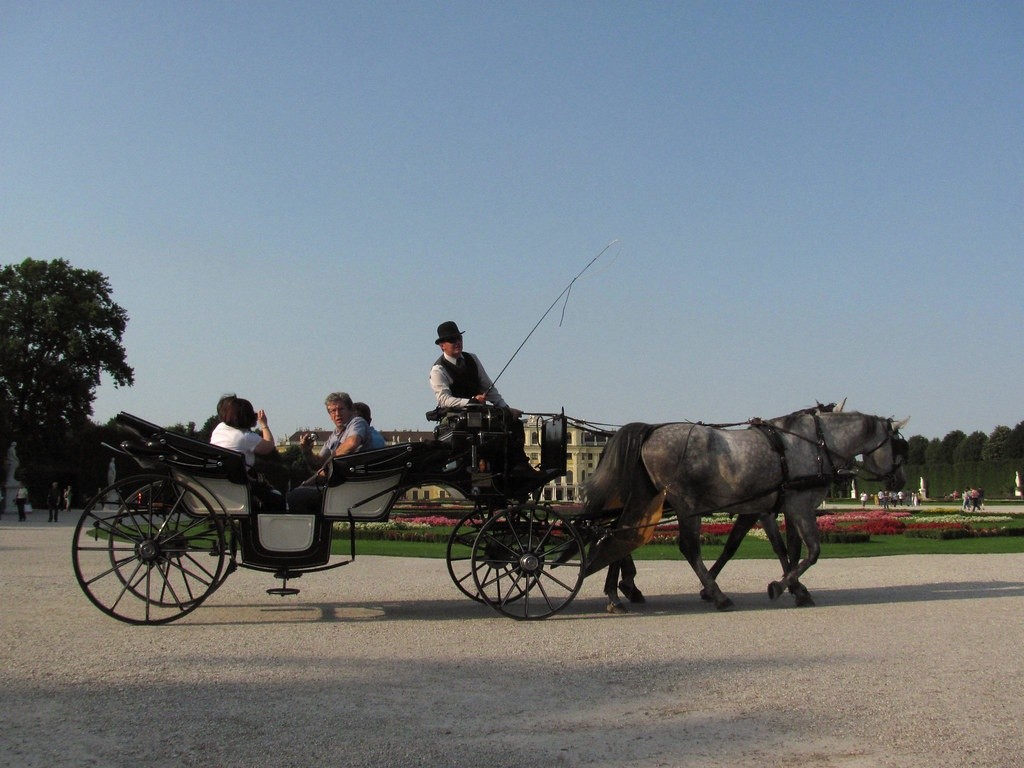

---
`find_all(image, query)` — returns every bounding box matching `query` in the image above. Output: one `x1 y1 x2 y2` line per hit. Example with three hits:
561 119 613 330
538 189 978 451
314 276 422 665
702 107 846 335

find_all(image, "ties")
457 357 465 368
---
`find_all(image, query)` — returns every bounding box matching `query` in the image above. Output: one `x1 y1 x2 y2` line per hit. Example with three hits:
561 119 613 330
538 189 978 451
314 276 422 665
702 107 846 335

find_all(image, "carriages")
71 399 911 625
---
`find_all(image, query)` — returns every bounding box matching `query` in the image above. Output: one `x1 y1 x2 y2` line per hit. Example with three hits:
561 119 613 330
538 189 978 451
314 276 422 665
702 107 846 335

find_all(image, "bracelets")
504 405 509 409
261 426 269 430
472 396 475 400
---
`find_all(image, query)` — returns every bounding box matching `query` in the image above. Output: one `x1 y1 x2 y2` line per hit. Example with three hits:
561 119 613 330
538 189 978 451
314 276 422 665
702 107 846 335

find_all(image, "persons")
429 321 536 473
210 390 386 513
109 458 116 486
6 442 19 482
14 481 29 522
46 482 61 523
60 486 72 512
859 485 988 514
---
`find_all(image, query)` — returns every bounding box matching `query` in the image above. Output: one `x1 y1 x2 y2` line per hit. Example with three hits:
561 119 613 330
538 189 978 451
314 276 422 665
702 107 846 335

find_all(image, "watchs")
302 481 306 486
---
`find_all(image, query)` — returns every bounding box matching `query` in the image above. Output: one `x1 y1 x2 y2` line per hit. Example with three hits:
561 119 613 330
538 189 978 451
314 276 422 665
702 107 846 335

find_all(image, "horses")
577 397 911 614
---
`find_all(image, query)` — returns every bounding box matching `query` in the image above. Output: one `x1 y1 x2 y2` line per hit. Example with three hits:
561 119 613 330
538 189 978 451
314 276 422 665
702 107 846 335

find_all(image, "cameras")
306 432 319 445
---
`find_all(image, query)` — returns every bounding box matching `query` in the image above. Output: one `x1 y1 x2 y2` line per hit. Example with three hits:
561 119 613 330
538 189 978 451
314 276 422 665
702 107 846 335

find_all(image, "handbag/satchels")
300 474 325 487
23 503 33 513
246 461 279 497
967 495 971 499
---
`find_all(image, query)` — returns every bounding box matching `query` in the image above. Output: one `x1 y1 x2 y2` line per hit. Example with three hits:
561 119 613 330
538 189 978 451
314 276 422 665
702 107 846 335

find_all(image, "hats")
435 321 465 345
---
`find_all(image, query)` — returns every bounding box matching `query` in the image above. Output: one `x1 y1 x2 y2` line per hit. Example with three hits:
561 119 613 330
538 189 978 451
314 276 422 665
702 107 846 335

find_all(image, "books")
851 470 1020 490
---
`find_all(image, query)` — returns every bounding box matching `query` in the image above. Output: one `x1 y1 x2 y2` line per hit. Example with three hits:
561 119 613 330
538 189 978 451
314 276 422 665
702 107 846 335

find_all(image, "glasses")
445 335 462 343
